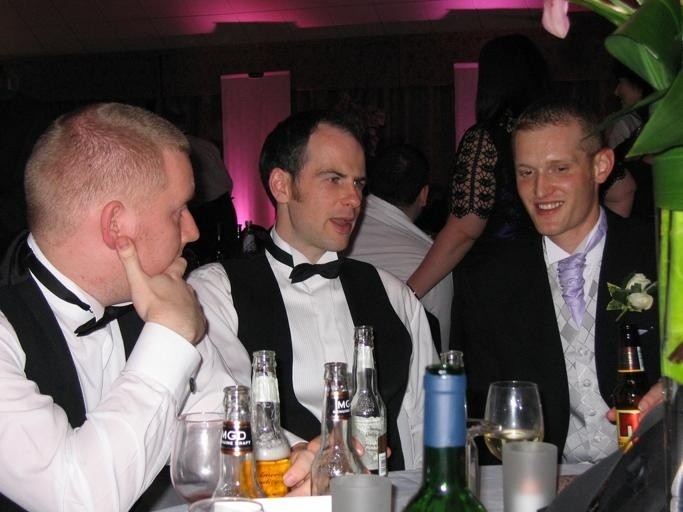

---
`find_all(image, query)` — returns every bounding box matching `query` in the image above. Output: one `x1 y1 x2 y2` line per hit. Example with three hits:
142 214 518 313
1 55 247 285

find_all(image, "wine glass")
482 378 545 458
168 411 224 504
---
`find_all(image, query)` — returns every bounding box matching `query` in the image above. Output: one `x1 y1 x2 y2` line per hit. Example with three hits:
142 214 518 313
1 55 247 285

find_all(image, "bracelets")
406 283 421 303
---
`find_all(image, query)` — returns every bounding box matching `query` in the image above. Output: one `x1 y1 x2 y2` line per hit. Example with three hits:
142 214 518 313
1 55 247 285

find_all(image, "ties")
557 254 585 328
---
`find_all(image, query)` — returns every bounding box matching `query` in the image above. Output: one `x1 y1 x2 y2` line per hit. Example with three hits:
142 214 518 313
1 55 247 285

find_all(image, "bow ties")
74 304 134 338
289 260 343 284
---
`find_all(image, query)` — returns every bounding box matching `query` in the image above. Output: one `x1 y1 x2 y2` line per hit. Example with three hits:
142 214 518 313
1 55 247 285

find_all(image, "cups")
499 440 558 511
187 497 264 512
330 472 395 512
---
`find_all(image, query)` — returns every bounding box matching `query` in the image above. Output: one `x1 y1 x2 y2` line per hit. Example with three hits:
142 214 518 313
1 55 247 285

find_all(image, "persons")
185 109 441 487
334 100 456 368
402 34 595 464
173 72 238 248
1 99 330 509
599 63 653 153
443 92 663 475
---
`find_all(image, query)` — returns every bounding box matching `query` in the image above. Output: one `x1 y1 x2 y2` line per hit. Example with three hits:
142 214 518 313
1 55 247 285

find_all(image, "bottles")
308 361 369 493
614 319 653 452
439 350 480 498
235 348 294 497
212 385 265 502
348 325 389 479
209 216 260 261
401 362 487 512
